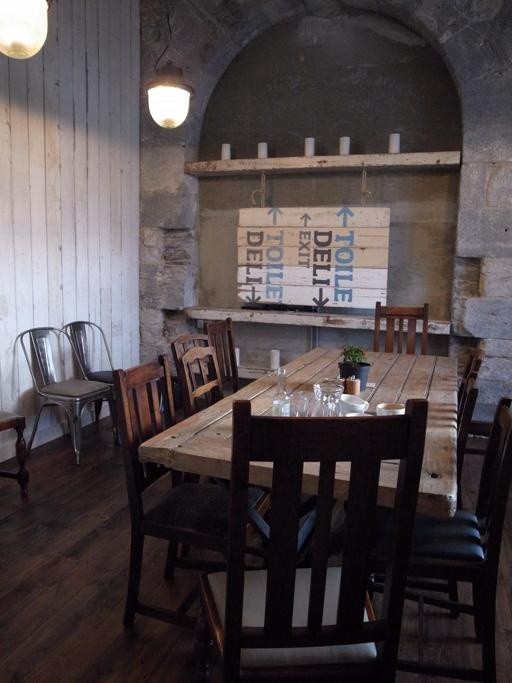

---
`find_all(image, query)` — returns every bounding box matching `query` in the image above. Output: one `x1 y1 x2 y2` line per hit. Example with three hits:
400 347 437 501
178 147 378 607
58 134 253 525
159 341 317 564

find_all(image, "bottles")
272 368 291 417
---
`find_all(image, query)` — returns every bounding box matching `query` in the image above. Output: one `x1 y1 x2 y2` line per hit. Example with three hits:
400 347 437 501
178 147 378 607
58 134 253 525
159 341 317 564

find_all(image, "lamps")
144 0 195 128
0 0 54 60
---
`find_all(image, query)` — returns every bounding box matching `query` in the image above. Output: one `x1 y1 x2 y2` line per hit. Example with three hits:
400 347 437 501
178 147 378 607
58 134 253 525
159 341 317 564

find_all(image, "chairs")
114 300 512 680
20 321 163 466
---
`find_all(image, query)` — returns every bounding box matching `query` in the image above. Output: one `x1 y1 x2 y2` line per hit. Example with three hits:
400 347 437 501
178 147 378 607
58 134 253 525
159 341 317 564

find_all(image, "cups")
270 350 280 370
295 378 406 418
